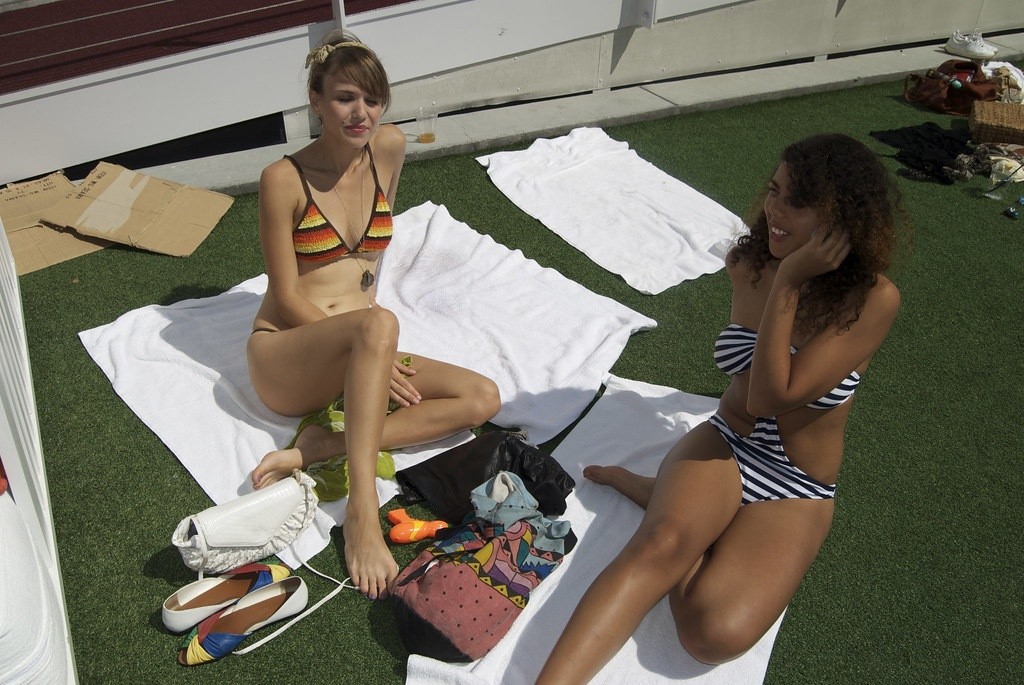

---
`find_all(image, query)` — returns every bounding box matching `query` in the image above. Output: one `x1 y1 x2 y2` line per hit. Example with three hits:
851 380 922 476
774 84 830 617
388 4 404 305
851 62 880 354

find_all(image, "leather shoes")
161 564 310 666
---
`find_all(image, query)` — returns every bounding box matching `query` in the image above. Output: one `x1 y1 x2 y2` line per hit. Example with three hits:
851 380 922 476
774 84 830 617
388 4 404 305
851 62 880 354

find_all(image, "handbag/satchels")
903 60 999 114
169 469 317 574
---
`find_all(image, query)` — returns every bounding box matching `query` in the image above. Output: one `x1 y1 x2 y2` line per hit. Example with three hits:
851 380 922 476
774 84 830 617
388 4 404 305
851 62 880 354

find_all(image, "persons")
246 29 502 596
533 133 902 685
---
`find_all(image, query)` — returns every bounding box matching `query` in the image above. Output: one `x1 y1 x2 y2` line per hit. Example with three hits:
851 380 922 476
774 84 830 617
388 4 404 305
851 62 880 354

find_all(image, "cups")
415 105 437 143
983 164 1016 200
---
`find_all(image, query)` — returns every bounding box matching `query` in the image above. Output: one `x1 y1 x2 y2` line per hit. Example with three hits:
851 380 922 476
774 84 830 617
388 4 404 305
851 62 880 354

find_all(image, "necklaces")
318 135 374 286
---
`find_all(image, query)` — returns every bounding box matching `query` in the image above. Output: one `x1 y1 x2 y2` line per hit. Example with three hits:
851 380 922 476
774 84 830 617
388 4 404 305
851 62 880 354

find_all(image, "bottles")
926 68 962 89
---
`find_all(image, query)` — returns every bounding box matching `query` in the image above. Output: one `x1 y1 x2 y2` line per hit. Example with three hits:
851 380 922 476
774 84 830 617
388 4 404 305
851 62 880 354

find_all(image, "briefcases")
970 100 1024 144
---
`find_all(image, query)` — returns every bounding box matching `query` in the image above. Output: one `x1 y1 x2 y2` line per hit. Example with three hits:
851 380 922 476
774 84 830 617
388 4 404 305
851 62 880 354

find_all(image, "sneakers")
944 30 998 59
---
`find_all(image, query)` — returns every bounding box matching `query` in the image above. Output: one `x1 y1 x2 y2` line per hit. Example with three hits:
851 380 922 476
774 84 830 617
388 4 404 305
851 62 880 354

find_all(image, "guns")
387 508 450 544
